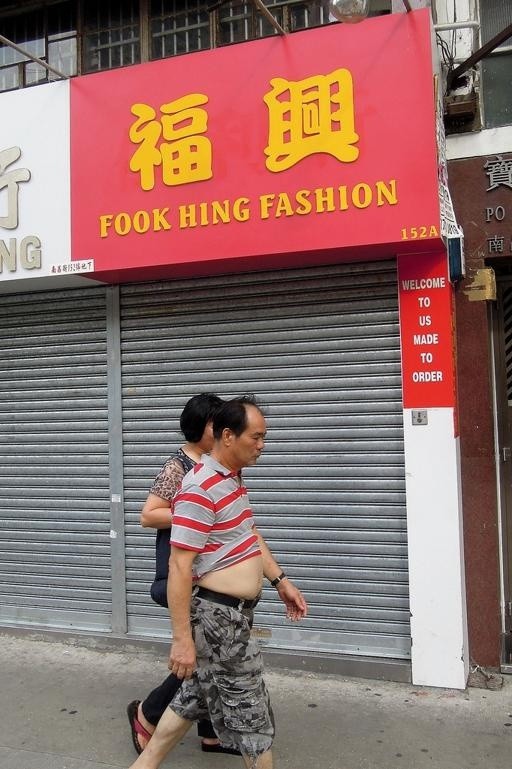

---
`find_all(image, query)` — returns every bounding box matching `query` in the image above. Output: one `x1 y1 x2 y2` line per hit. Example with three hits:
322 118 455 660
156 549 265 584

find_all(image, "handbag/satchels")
149 523 171 608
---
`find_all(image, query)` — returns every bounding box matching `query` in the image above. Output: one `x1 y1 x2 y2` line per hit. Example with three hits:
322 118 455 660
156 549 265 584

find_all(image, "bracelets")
270 572 286 584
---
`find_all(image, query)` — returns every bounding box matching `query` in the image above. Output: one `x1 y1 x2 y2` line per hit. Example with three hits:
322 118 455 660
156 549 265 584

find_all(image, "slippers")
200 736 242 754
126 698 151 755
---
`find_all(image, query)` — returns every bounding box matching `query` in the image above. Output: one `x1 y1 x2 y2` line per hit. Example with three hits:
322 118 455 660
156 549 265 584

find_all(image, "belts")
190 583 263 609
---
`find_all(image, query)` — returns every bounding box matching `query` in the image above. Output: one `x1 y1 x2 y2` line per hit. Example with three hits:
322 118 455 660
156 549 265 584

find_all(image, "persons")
124 393 308 768
128 393 230 758
